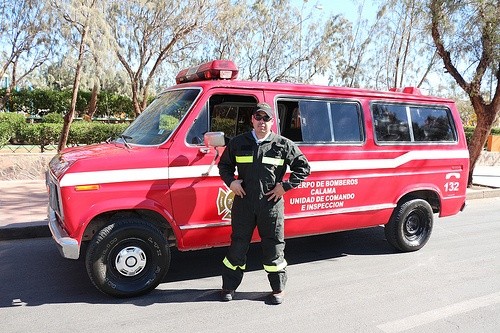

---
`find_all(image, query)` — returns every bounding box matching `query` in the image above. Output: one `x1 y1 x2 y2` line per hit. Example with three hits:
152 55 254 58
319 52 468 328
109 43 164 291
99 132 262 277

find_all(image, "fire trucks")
44 60 470 299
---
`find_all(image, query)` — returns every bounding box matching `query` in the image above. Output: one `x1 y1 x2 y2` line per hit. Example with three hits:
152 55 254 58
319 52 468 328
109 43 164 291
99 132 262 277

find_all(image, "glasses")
254 114 272 122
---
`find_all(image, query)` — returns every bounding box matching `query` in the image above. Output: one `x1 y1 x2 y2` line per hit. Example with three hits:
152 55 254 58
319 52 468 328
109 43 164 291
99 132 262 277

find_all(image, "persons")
218 103 311 305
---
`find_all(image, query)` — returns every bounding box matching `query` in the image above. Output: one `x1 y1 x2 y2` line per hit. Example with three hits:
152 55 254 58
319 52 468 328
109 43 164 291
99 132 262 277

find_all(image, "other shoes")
221 288 234 301
270 291 284 304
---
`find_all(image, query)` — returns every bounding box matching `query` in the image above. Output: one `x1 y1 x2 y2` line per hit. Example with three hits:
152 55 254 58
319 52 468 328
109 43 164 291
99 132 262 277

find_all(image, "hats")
252 102 273 111
